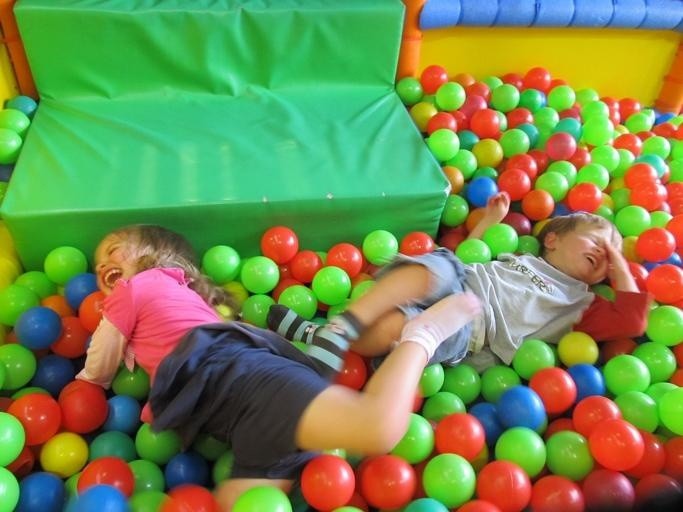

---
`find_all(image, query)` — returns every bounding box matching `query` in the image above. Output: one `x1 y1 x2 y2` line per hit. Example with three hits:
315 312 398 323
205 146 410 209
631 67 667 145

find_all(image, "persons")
267 212 648 380
75 225 482 511
